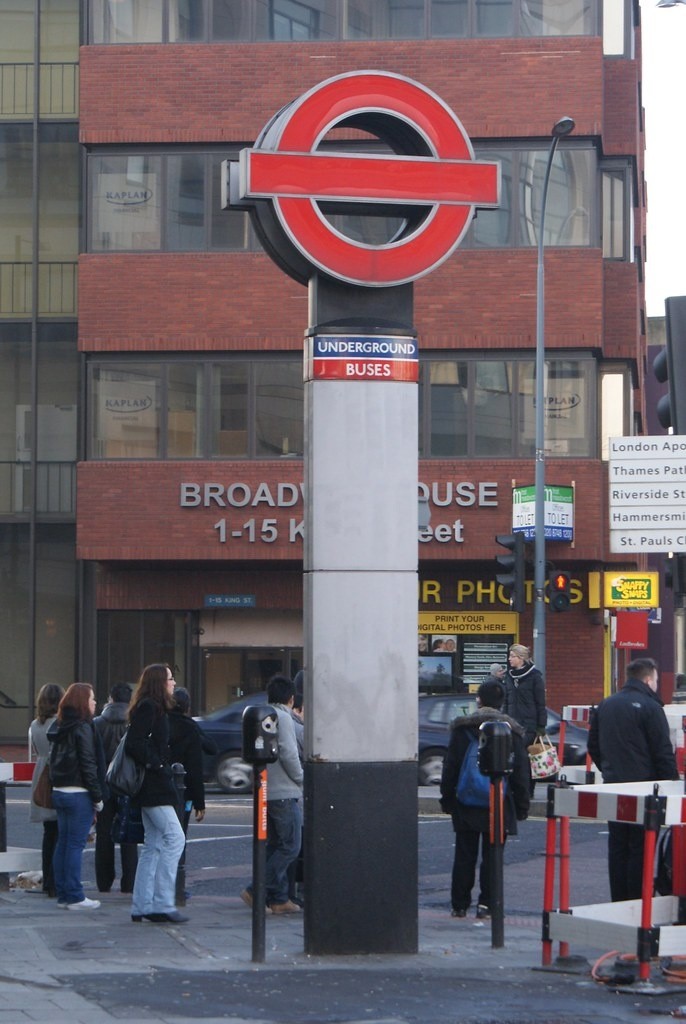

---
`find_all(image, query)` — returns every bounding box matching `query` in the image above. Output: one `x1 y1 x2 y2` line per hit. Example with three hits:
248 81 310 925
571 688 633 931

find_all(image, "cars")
189 689 269 793
418 693 589 786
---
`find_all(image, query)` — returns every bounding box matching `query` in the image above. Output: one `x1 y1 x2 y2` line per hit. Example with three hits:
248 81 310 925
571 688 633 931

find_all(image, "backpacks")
653 825 674 897
454 728 507 807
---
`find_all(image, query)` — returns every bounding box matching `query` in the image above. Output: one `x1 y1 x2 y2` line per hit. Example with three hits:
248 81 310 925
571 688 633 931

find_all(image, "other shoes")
67 897 100 910
57 901 68 908
47 890 58 897
476 904 505 919
451 908 466 918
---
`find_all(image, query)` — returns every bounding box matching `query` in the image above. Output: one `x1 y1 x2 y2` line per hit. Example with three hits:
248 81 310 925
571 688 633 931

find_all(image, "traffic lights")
549 571 571 612
654 295 686 435
665 553 686 596
494 533 525 613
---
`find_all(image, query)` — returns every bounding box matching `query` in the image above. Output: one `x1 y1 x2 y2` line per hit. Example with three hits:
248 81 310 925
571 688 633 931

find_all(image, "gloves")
536 725 546 737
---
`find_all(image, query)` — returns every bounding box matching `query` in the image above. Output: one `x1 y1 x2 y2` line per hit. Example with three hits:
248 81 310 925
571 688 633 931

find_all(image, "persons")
587 657 681 902
30 683 65 899
92 683 143 893
125 663 189 924
490 663 503 679
501 644 548 799
440 679 531 921
166 687 205 908
240 669 305 916
417 634 456 652
47 682 103 912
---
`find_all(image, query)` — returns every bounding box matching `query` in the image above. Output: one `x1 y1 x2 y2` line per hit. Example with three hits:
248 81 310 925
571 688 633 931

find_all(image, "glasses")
510 654 520 658
165 677 174 680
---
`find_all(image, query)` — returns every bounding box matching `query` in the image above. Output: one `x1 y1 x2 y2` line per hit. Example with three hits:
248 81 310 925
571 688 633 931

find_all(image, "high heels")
150 911 189 923
131 911 167 922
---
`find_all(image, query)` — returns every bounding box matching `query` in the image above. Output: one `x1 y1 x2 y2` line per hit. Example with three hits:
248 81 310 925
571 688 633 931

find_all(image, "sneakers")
270 899 300 914
240 889 272 915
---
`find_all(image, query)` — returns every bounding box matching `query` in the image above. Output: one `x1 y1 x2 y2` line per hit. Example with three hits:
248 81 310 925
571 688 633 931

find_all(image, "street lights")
531 118 577 682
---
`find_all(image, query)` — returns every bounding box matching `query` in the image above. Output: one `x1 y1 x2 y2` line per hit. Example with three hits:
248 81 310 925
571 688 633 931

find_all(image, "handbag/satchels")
110 795 146 844
105 732 144 797
527 735 561 779
32 762 55 810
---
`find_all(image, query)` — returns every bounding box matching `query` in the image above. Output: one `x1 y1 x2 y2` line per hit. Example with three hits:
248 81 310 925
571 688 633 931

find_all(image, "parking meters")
243 704 280 966
